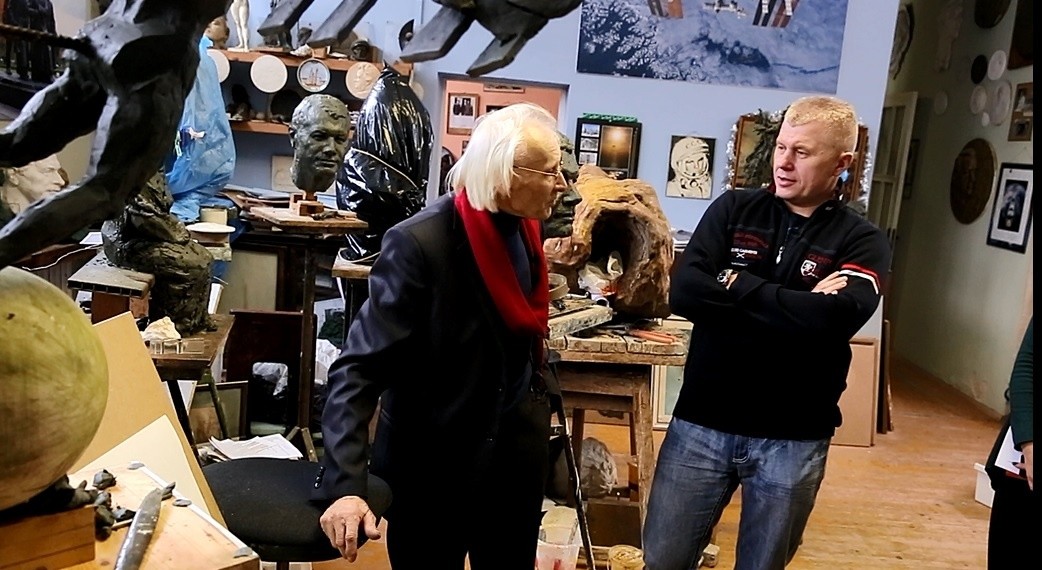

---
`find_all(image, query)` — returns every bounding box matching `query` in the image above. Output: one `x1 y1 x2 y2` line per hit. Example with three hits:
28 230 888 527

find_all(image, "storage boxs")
584 499 643 550
974 463 996 509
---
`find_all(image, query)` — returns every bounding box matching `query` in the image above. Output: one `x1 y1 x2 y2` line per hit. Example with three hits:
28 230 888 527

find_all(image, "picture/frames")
730 115 867 205
987 162 1033 254
446 92 480 135
902 138 921 200
484 83 526 93
1007 83 1033 141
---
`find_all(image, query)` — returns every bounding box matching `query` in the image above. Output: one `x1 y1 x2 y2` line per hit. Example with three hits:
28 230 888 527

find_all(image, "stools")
201 458 389 570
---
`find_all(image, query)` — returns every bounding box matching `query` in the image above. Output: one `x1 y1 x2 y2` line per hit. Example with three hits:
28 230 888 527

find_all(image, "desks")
252 208 368 464
144 313 236 465
331 248 374 348
548 331 688 558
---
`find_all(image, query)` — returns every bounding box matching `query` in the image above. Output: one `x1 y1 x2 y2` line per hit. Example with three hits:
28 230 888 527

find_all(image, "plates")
297 58 330 93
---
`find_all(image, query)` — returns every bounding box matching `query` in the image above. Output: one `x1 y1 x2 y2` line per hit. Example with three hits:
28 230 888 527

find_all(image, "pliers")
611 330 676 343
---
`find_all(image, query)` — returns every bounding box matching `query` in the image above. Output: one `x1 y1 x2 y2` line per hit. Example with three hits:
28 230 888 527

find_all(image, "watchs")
717 268 738 287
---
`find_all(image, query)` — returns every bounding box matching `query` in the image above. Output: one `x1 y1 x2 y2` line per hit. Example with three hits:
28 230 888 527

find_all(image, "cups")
534 523 582 570
200 207 227 225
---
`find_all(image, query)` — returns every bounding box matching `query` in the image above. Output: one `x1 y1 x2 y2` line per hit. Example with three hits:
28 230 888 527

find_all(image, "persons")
984 313 1033 570
288 94 351 193
0 154 65 215
205 16 230 50
0 0 234 269
230 0 249 50
321 102 568 570
642 95 890 570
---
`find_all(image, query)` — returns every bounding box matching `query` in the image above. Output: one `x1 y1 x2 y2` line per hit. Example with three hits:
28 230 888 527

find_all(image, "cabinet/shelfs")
219 49 415 134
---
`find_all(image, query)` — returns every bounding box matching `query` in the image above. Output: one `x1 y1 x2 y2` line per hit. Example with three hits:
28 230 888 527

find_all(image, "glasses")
513 157 564 180
